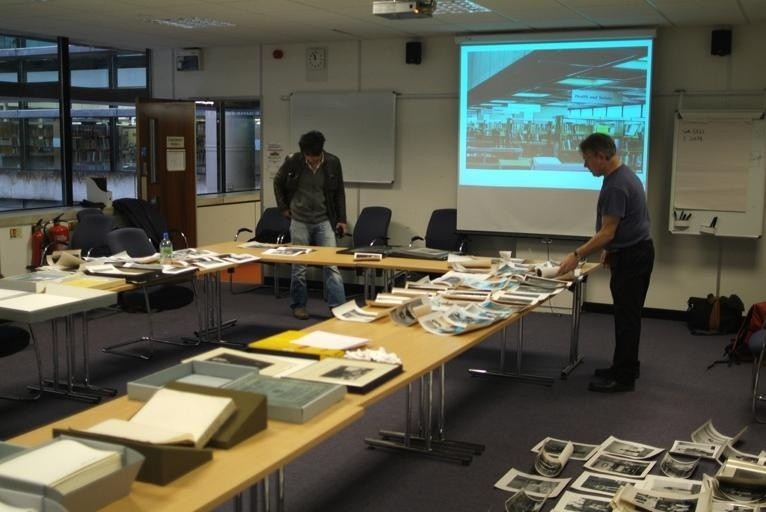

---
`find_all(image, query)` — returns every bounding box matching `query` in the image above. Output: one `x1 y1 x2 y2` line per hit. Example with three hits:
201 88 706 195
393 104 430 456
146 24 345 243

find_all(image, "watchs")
574 249 582 262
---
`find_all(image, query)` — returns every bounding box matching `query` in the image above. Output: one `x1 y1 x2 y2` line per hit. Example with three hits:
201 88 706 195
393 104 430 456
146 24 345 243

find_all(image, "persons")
557 133 654 394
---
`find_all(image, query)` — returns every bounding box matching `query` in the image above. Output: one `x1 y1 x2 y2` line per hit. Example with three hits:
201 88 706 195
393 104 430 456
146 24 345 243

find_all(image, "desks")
192 239 601 376
0 241 260 405
249 272 572 464
0 363 365 512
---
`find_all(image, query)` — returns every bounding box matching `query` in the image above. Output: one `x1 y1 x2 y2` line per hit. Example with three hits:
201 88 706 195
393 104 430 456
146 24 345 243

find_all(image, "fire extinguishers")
43 213 69 251
30 219 45 265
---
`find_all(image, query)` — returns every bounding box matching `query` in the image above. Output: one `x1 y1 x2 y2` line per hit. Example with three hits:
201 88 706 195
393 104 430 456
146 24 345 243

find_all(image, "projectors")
371 0 436 22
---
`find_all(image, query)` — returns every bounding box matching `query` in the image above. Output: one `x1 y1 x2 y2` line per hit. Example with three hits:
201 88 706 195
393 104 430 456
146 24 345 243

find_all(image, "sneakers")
293 306 310 319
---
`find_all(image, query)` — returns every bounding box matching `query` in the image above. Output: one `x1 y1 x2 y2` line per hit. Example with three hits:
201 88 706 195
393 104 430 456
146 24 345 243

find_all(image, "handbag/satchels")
686 294 743 337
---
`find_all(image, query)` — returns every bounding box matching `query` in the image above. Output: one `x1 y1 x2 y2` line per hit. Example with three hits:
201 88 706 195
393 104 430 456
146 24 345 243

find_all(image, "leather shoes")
588 379 634 394
595 361 640 380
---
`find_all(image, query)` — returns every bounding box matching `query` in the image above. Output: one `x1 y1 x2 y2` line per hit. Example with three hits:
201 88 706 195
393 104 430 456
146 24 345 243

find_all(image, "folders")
387 248 457 261
336 246 392 259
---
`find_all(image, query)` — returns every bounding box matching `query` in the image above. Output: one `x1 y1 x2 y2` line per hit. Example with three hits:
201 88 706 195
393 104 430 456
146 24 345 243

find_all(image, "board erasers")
710 216 717 227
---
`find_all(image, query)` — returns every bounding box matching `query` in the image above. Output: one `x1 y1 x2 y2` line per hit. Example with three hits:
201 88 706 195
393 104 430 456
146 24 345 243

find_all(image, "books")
273 129 347 320
68 386 238 450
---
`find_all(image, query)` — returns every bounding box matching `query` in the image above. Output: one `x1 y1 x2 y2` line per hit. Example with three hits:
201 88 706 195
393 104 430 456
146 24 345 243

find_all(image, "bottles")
158 233 174 272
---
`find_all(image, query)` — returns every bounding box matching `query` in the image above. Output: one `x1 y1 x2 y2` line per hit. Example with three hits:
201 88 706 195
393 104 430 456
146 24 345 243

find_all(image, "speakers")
711 29 731 56
405 41 422 64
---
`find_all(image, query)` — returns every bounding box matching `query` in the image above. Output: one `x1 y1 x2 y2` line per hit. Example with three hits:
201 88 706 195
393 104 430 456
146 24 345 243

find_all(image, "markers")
679 211 684 220
685 214 691 220
674 211 678 220
682 214 686 220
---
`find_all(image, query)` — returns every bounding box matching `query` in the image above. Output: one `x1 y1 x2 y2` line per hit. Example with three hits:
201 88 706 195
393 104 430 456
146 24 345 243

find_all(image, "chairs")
749 318 766 422
40 216 122 326
337 207 392 300
230 207 294 298
407 210 471 279
102 228 199 362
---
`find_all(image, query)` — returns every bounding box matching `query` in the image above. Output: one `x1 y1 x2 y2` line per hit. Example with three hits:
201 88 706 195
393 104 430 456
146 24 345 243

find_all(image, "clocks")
304 45 327 82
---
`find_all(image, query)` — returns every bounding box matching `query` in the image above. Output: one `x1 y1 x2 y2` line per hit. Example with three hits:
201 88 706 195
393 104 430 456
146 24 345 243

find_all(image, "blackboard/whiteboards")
287 87 397 184
669 110 762 239
